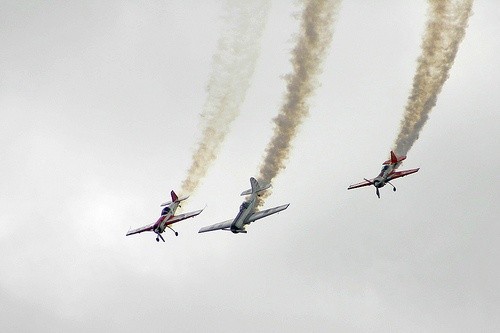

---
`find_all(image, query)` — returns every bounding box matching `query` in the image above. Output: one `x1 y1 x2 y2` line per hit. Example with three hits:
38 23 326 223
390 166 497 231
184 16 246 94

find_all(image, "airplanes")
197 177 291 233
348 151 420 198
126 190 207 242
